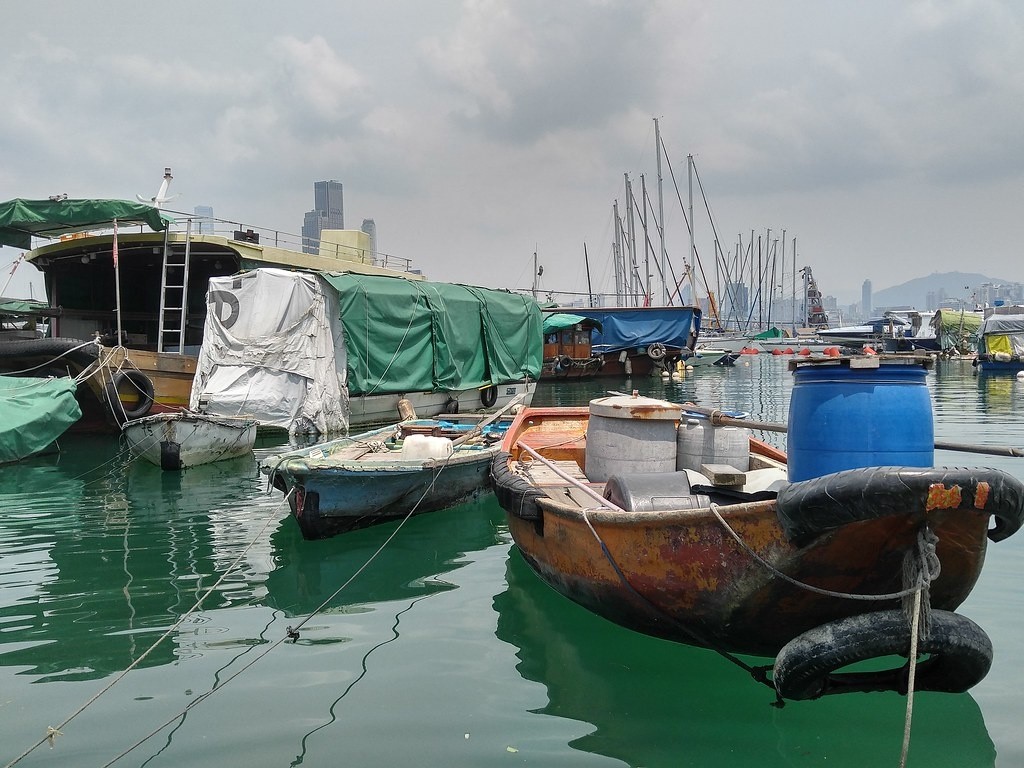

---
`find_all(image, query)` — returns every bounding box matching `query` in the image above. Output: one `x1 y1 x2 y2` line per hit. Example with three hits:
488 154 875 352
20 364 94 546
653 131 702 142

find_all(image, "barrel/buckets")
829 347 839 357
783 348 794 354
798 348 810 355
584 414 677 489
677 416 750 480
772 348 783 355
740 348 759 355
823 348 830 354
786 365 935 488
864 347 877 355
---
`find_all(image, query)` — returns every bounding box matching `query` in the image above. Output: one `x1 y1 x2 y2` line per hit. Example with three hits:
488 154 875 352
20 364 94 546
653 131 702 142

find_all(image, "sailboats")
694 228 844 353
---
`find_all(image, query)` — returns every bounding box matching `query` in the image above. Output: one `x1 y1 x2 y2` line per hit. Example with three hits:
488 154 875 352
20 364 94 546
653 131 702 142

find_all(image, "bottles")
400 434 454 461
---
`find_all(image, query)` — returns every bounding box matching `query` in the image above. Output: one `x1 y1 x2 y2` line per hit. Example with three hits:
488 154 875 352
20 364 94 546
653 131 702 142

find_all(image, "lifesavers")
898 339 907 348
481 384 498 407
972 357 981 367
770 607 995 701
445 399 459 424
671 354 682 363
104 369 154 418
560 356 571 369
648 343 667 365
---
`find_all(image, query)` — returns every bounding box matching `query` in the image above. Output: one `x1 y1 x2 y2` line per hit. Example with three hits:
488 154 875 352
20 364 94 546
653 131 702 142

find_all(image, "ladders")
157 219 192 356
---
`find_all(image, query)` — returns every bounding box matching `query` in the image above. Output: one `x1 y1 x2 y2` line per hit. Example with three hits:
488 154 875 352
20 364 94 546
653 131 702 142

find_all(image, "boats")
0 374 83 464
1 168 543 434
549 306 702 375
975 314 1024 370
541 311 603 380
487 389 1024 700
260 418 512 541
121 406 261 469
684 344 741 368
816 310 947 352
